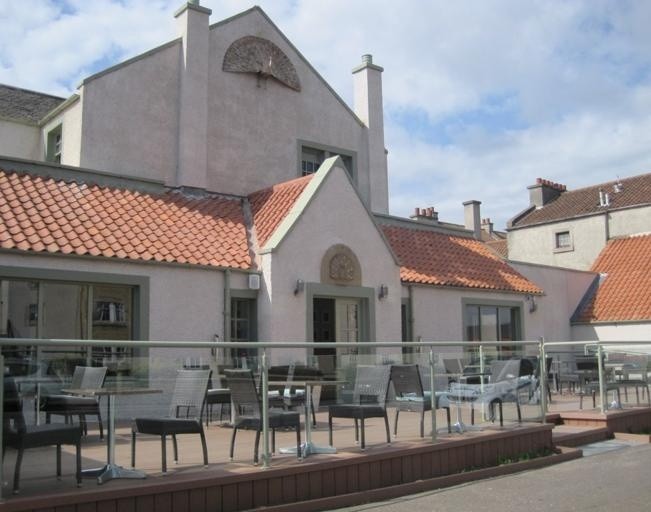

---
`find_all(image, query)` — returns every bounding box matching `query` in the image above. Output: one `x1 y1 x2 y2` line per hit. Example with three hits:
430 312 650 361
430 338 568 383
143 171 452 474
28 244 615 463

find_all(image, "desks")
268 381 352 459
62 388 163 485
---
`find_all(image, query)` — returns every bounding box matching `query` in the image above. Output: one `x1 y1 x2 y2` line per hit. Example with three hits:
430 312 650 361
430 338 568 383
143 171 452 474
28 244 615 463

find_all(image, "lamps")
378 283 389 300
294 278 306 296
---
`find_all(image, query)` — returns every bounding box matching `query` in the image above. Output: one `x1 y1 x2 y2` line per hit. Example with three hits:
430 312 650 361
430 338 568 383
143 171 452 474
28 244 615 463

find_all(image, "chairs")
389 359 522 439
2 375 85 494
185 366 324 462
506 351 651 410
35 366 108 439
328 364 394 449
131 370 211 472
